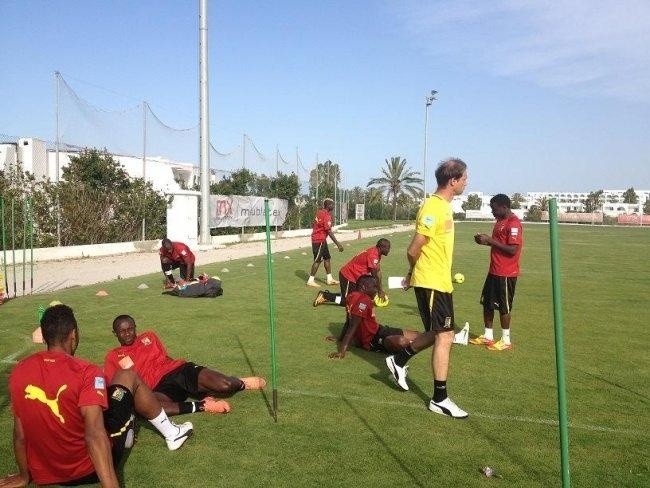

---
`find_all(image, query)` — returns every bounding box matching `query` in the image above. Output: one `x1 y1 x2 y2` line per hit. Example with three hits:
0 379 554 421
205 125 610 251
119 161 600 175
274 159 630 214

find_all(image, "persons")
103 314 266 416
314 238 390 307
385 157 469 419
159 238 195 284
326 275 470 359
306 198 344 288
0 304 194 488
467 194 522 351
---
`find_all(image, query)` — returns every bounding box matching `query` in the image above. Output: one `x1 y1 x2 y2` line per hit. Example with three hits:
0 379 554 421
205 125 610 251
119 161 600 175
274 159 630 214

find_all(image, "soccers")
454 273 464 283
375 293 388 306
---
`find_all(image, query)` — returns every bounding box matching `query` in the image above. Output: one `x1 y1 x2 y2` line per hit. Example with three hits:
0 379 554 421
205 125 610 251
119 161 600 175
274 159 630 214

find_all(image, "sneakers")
385 355 410 391
202 396 230 414
326 280 340 285
314 291 327 307
239 376 266 390
486 340 512 350
470 334 495 345
428 397 468 419
458 322 469 345
305 280 321 287
166 421 194 451
124 428 136 449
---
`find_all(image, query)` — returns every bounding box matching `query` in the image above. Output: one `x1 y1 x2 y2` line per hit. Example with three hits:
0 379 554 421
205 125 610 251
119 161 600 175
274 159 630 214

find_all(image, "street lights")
422 87 438 203
